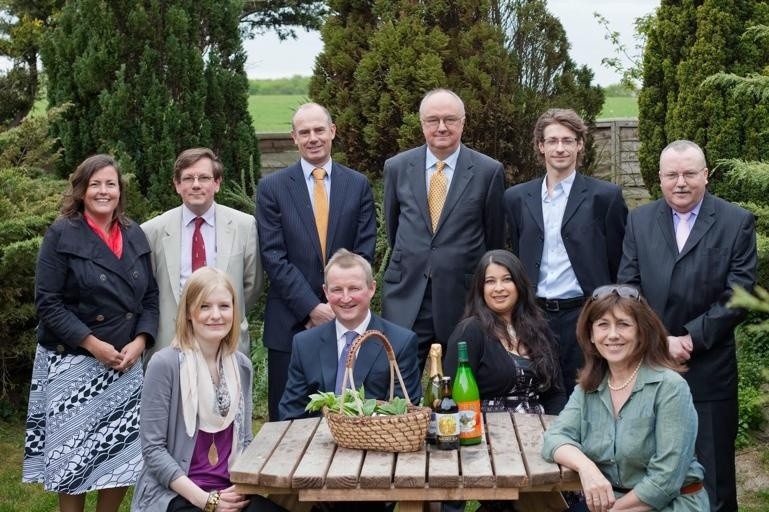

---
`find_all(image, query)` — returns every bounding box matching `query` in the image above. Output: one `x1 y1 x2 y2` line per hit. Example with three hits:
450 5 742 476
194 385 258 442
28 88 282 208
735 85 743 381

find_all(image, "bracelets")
203 488 221 511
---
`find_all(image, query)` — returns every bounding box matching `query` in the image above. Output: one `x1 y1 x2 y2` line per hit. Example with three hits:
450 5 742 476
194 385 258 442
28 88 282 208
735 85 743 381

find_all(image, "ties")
427 162 447 233
334 333 359 397
311 168 330 270
675 213 692 251
191 216 206 272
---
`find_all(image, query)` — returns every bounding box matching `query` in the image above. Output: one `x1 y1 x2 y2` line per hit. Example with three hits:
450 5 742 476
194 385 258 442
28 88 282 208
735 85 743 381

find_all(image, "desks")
231 409 584 511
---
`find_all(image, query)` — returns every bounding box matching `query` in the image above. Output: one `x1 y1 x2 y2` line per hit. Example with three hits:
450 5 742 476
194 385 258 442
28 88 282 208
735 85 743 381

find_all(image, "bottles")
423 341 444 445
434 375 460 450
452 341 484 448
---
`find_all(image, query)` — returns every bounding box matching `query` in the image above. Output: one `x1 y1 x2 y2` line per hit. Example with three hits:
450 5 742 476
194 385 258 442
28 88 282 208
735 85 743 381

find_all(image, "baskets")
322 330 431 453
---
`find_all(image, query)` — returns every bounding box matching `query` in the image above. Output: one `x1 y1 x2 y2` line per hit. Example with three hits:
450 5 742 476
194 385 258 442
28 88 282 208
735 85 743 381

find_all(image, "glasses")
591 286 640 302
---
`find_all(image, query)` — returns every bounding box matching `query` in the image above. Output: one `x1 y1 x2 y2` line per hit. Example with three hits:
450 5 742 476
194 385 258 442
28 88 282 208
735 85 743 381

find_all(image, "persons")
138 145 262 458
615 137 757 512
276 247 421 511
21 153 159 512
443 249 567 511
538 285 713 511
381 88 507 373
128 263 291 511
254 101 378 420
500 106 628 394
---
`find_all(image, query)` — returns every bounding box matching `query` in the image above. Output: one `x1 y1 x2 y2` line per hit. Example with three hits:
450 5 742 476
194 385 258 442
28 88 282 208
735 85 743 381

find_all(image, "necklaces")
607 356 645 391
206 351 223 387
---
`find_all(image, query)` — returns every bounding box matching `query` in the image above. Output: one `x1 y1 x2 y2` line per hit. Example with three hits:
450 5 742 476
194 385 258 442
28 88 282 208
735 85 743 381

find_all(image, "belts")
538 297 587 311
